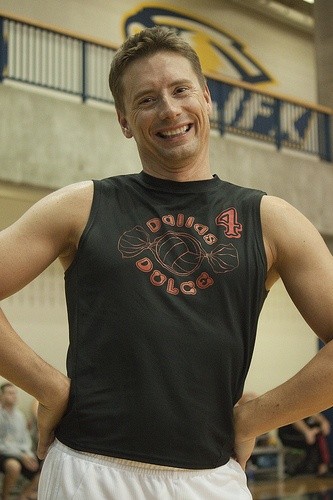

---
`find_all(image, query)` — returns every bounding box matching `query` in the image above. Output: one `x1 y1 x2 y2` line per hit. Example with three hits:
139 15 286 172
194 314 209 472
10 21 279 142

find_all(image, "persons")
277 413 330 476
0 382 42 500
0 26 332 499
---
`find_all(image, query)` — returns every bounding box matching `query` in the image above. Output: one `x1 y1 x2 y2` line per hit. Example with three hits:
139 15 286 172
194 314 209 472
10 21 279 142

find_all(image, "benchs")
246 446 305 483
